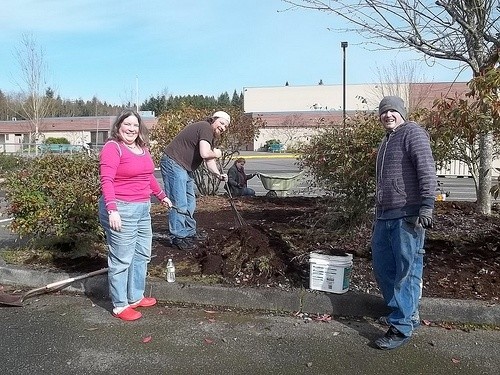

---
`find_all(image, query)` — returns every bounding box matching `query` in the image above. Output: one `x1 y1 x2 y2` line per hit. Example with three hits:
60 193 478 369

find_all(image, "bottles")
167 259 175 283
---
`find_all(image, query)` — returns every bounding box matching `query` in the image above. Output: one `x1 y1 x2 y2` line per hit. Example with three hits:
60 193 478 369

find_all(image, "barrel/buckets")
309 251 354 294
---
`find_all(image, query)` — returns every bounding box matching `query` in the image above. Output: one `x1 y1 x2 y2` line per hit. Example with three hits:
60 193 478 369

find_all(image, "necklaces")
126 145 136 148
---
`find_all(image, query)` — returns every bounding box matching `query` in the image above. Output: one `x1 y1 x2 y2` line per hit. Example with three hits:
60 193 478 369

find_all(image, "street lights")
341 40 348 127
135 73 138 114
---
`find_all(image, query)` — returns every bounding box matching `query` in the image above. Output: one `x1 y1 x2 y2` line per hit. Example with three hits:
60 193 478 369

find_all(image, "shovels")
0 268 108 306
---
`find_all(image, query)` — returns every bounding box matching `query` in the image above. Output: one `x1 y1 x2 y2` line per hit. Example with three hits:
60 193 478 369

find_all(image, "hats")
379 96 407 121
212 110 230 124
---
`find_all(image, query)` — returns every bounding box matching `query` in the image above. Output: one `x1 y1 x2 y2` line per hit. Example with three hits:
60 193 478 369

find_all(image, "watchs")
107 210 113 214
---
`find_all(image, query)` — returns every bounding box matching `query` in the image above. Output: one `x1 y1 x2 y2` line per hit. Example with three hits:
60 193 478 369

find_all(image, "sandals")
129 297 156 308
112 306 142 320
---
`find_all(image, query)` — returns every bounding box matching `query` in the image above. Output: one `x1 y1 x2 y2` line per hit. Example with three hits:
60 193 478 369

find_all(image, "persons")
159 111 231 250
370 95 436 351
99 109 173 321
224 158 259 196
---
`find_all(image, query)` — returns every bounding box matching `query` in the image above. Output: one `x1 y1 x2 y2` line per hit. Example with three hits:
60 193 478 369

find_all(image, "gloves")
238 183 243 189
418 206 433 228
253 171 258 176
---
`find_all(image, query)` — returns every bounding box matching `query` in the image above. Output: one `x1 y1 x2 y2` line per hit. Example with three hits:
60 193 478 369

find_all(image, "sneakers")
172 237 197 251
185 233 206 242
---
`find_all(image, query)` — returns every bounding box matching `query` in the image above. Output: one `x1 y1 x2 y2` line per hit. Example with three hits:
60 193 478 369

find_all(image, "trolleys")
256 169 306 197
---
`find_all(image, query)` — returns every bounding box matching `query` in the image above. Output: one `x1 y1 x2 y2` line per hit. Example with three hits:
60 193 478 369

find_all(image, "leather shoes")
374 325 411 350
378 315 420 328
